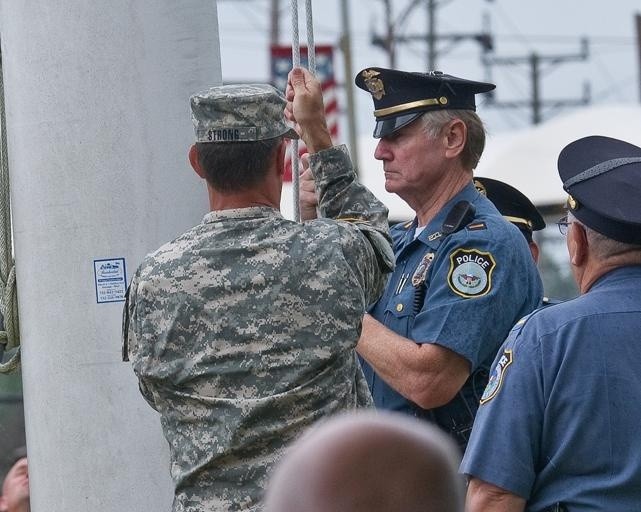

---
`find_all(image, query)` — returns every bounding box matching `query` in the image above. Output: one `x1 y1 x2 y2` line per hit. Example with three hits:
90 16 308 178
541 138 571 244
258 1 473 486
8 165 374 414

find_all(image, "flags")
271 45 339 182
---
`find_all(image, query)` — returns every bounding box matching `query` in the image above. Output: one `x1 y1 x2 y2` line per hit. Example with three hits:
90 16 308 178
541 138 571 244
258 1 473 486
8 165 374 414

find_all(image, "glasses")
555 215 589 247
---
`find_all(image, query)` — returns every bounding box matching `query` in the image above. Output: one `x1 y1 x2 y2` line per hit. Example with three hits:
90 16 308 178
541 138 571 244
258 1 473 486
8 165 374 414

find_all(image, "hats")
356 67 496 138
558 136 641 244
189 84 300 143
473 177 546 242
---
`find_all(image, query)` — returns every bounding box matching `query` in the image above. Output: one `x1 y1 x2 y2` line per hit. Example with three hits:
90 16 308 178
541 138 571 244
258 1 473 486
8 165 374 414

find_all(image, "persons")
457 135 639 510
120 66 396 511
296 67 544 462
298 151 563 308
262 408 466 511
0 454 30 512
